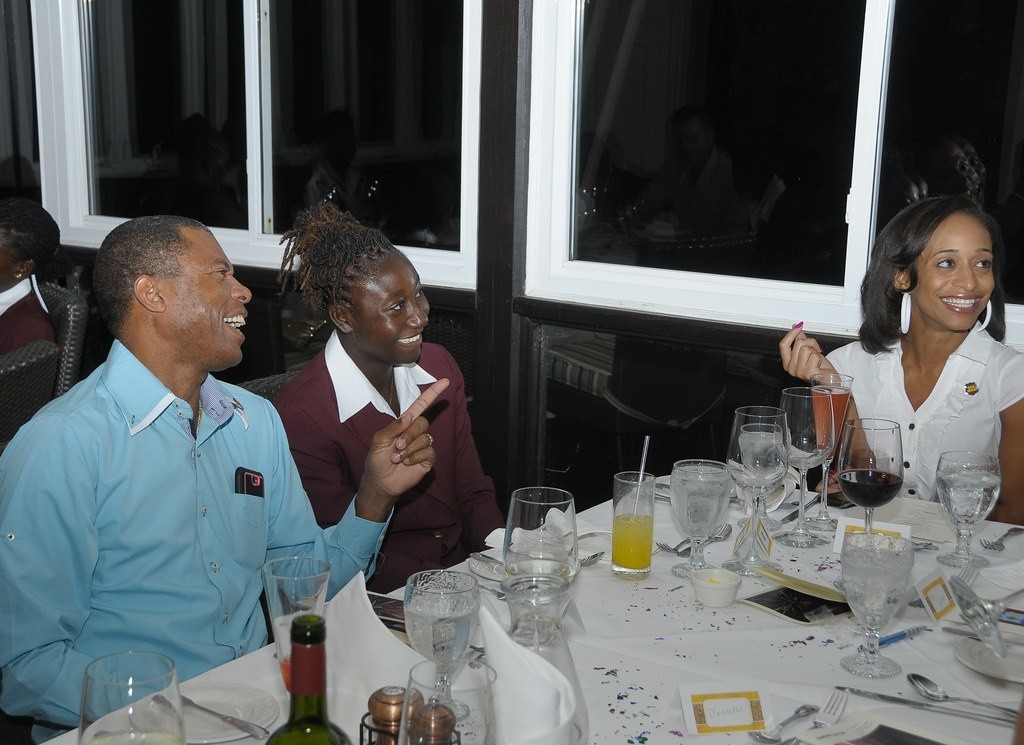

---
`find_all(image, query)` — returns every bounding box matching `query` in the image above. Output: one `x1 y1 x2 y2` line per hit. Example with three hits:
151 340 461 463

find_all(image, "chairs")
0 280 88 456
209 289 314 402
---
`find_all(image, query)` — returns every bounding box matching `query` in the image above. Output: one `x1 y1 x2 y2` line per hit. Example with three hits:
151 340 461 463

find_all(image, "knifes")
777 490 824 525
834 685 1018 728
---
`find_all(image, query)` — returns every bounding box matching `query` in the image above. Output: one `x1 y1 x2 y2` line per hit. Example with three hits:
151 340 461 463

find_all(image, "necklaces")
196 399 201 426
597 163 615 185
389 376 394 406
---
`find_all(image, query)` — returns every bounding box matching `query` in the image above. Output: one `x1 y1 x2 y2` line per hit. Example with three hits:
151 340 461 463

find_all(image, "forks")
909 564 977 607
784 690 848 745
979 525 1023 553
654 538 690 554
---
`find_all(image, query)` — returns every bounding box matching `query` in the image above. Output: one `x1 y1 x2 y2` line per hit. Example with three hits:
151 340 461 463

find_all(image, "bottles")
264 616 354 745
499 574 590 743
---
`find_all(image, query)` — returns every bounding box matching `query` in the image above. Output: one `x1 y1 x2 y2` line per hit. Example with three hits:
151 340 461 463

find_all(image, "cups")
77 651 189 745
502 487 577 590
263 557 332 709
610 472 656 581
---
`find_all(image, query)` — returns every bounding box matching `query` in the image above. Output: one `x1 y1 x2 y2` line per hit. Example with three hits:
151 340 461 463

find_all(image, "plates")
955 630 1023 686
469 540 580 586
127 682 279 745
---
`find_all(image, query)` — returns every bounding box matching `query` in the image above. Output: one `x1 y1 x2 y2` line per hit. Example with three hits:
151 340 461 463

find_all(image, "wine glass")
668 368 1024 670
397 658 499 745
405 569 481 723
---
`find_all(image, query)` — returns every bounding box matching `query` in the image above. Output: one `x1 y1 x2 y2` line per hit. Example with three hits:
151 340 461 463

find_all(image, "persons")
778 195 1024 528
0 197 61 355
300 110 390 230
574 334 725 441
136 110 246 227
0 214 450 745
274 193 504 597
582 127 647 221
627 105 753 226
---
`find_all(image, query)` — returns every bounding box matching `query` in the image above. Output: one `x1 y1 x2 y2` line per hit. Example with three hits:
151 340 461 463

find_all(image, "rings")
425 433 434 446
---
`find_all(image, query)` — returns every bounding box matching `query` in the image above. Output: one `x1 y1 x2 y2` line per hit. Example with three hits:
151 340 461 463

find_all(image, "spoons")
905 673 1018 718
748 702 821 744
576 552 604 570
677 521 731 559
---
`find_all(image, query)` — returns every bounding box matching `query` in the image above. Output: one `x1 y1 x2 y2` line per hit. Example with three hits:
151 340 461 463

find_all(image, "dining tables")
38 466 1024 745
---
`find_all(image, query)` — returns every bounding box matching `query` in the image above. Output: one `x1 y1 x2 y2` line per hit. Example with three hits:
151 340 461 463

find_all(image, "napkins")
485 527 573 561
310 569 575 745
729 460 808 513
971 560 1024 601
